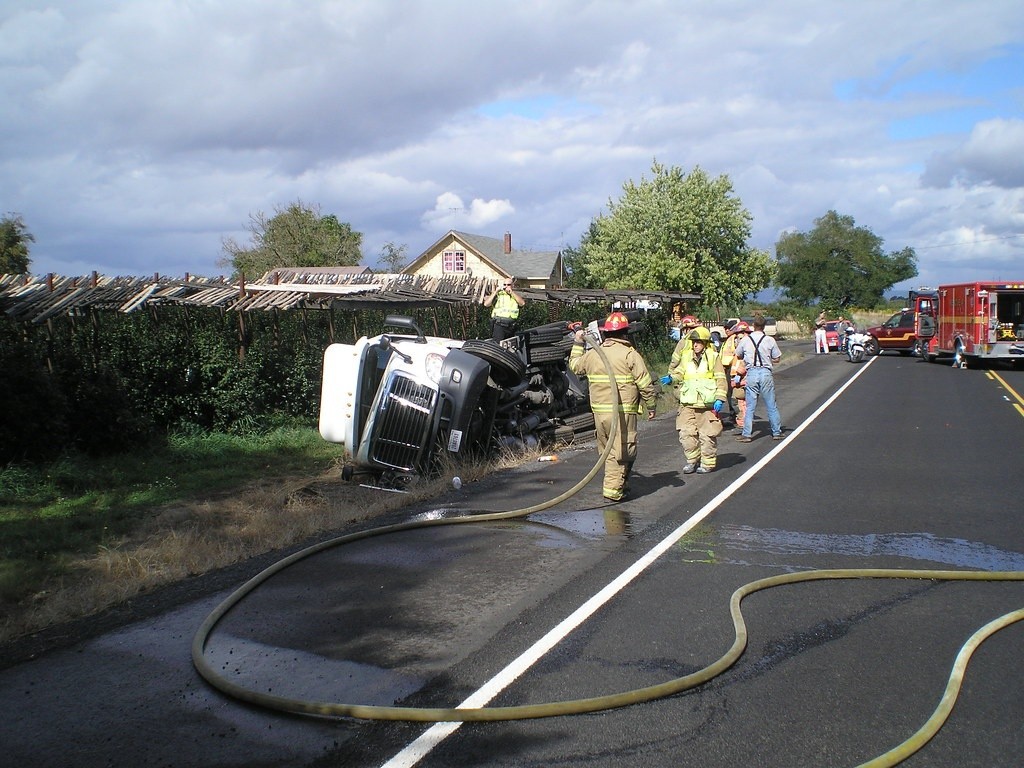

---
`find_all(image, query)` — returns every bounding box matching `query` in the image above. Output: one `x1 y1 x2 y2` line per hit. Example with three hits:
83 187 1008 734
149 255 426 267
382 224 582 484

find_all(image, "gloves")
660 375 672 385
714 399 723 412
734 376 740 384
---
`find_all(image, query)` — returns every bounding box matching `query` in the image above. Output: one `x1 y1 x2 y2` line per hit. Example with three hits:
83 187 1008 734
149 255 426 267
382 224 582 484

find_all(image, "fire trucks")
912 281 1024 371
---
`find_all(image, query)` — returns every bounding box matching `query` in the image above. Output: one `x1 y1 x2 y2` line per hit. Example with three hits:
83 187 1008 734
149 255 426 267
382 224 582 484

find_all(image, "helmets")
599 312 630 331
679 315 701 329
846 326 854 334
689 327 715 343
732 322 753 334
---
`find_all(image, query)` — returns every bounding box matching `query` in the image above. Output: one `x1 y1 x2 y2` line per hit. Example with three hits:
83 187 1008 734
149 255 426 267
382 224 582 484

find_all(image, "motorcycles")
847 317 873 364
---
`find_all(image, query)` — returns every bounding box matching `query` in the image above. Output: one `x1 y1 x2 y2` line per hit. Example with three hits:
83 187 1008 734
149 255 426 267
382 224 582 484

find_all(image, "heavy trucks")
319 307 647 496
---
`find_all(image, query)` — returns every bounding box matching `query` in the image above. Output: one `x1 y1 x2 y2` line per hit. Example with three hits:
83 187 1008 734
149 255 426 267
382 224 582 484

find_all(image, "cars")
814 320 853 353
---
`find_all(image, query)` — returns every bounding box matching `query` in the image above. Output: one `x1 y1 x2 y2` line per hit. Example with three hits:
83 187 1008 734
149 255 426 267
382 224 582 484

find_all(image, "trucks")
708 315 777 342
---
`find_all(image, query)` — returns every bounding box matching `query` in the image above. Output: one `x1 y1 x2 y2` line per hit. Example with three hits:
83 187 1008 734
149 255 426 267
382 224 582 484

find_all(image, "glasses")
503 284 511 286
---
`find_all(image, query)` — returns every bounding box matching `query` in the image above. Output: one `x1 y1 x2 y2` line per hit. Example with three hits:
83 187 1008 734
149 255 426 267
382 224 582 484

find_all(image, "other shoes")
773 433 786 439
696 467 715 474
735 435 751 442
604 489 630 503
731 428 742 435
683 462 700 474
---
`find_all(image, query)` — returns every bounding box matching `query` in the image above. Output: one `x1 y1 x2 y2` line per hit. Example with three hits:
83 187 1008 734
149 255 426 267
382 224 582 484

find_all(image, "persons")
735 318 785 442
569 313 656 502
660 328 728 472
669 316 717 373
484 279 525 343
715 324 754 435
815 314 830 354
836 316 847 354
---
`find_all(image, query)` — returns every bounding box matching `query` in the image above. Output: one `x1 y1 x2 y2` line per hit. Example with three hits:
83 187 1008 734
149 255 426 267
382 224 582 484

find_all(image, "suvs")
858 311 923 358
